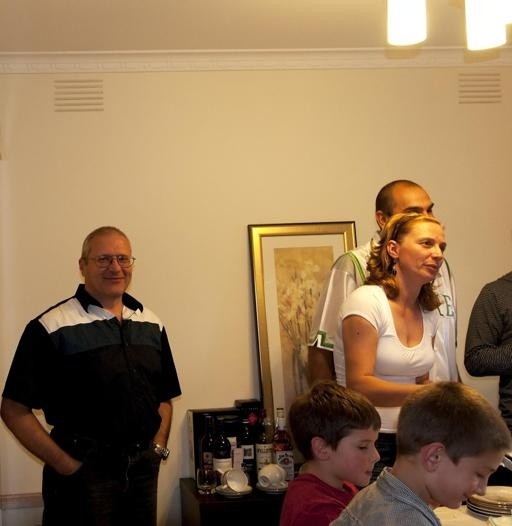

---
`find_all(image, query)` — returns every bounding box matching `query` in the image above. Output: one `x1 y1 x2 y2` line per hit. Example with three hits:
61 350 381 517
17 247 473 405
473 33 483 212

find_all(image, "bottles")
257 413 273 472
200 415 213 477
213 415 233 475
273 408 297 481
238 418 255 483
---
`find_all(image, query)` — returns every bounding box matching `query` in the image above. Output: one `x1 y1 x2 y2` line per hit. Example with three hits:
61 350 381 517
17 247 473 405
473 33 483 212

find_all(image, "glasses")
82 254 136 268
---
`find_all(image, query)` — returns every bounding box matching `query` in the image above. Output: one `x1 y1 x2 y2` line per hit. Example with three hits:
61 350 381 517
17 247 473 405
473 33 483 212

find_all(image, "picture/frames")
247 220 357 472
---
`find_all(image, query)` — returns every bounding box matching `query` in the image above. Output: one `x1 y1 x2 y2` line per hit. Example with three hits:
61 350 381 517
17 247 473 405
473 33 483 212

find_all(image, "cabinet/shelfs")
179 476 286 526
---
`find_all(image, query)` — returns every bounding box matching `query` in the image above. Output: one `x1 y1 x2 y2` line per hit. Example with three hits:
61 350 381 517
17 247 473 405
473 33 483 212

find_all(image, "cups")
197 468 216 494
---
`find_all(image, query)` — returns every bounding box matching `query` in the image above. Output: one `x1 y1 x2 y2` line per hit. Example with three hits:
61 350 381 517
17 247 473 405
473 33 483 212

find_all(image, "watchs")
152 442 170 461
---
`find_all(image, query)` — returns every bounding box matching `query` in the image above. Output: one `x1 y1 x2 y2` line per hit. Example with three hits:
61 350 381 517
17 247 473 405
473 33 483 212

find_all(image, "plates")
465 486 512 518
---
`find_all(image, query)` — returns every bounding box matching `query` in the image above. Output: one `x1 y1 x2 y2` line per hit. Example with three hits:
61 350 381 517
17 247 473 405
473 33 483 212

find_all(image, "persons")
465 272 512 486
327 383 512 526
332 213 448 484
305 180 464 389
0 227 183 526
279 378 381 526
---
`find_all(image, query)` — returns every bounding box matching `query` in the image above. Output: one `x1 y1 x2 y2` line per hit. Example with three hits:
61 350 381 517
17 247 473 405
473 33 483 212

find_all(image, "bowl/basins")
224 468 249 493
257 464 286 489
